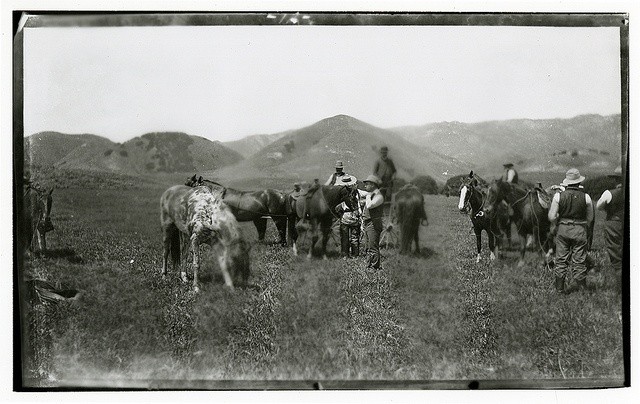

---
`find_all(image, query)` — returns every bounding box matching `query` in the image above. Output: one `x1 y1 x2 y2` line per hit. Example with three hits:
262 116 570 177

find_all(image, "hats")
336 174 357 186
608 166 622 178
363 175 383 189
378 147 389 152
333 161 346 168
563 168 585 185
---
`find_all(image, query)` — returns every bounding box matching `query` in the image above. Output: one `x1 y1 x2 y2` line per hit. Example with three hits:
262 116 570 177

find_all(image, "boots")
575 277 586 295
556 276 564 293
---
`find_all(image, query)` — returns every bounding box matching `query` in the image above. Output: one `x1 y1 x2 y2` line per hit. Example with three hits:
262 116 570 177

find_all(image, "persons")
361 175 384 270
596 166 624 293
323 161 346 186
503 163 518 186
547 168 595 295
372 146 396 202
335 175 361 258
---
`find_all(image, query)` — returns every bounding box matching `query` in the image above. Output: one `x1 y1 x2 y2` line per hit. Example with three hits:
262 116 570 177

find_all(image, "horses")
185 174 288 248
483 175 595 267
457 178 512 264
159 185 252 298
23 184 55 259
284 183 359 260
458 169 535 250
395 183 423 257
